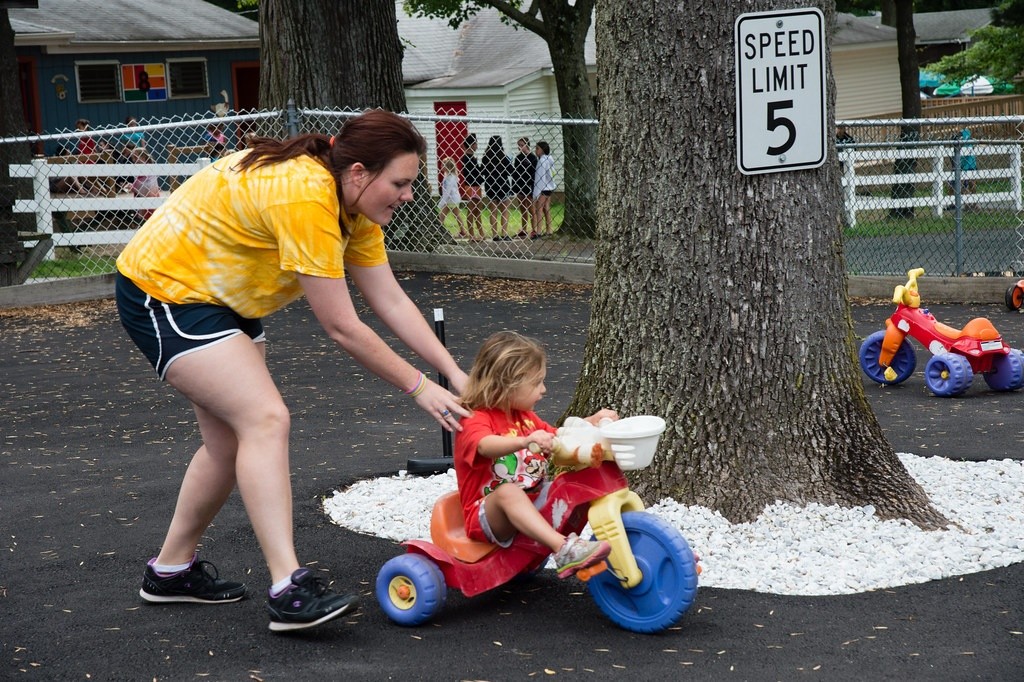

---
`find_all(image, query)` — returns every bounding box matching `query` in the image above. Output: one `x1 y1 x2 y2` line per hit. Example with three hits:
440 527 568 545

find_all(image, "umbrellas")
919 67 1016 99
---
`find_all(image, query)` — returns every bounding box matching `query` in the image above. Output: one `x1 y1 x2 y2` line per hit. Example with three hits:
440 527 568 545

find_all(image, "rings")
442 409 450 416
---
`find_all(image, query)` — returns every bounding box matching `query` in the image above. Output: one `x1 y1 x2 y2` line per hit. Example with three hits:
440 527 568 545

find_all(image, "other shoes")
529 232 553 241
469 237 486 243
459 230 470 237
493 235 512 241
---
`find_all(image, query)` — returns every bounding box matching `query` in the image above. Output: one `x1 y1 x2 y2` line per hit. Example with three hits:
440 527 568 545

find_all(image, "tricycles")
1005 280 1024 310
375 414 700 634
858 268 1024 397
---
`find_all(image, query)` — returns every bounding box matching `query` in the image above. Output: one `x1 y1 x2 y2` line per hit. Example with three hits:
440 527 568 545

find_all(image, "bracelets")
405 370 427 398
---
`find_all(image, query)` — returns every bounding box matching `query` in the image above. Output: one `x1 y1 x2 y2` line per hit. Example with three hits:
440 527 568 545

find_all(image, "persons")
114 110 471 632
944 129 979 211
453 333 701 578
835 127 859 168
438 134 556 242
53 104 257 227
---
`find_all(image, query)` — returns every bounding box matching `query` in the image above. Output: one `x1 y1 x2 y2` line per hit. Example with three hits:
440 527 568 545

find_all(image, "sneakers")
139 553 248 603
269 568 359 630
512 231 528 239
555 533 611 579
